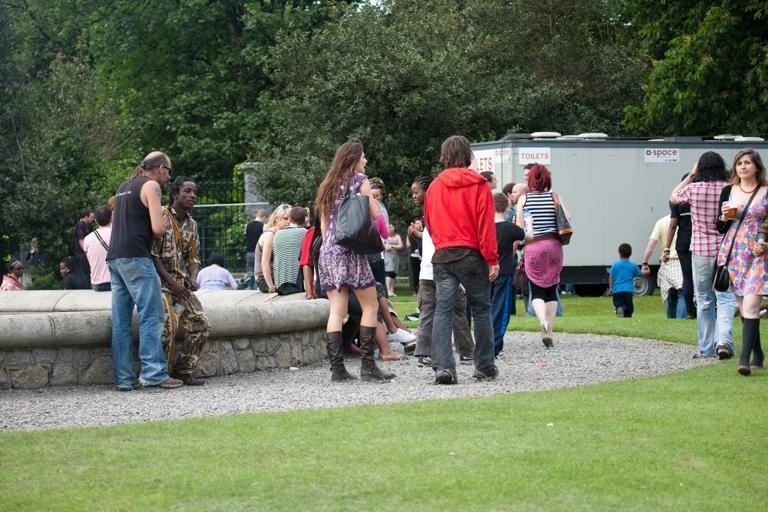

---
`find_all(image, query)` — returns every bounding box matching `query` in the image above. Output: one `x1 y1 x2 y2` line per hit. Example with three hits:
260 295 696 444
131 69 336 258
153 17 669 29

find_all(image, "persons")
74 211 94 272
659 173 697 319
716 150 768 376
197 254 239 290
0 258 24 291
105 151 184 391
83 206 112 291
151 176 211 386
641 214 683 319
753 242 768 257
245 136 570 383
609 244 648 317
670 152 738 360
107 196 115 222
60 256 91 289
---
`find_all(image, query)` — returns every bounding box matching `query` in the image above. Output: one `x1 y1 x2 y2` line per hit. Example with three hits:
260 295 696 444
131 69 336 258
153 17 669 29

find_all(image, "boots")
359 326 395 382
326 331 358 381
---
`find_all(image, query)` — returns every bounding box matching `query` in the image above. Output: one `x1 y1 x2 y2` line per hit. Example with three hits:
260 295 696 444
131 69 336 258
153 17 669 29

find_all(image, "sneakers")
472 366 498 382
416 356 431 367
459 354 474 365
157 371 204 389
387 329 415 355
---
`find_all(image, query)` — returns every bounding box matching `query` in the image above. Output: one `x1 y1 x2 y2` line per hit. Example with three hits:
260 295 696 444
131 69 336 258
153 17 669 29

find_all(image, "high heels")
541 322 552 347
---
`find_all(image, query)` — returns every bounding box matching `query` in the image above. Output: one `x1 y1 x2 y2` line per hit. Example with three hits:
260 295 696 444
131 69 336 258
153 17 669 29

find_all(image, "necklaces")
739 183 755 193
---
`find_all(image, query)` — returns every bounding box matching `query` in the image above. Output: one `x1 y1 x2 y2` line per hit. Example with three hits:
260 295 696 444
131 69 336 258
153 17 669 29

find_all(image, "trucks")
467 132 768 298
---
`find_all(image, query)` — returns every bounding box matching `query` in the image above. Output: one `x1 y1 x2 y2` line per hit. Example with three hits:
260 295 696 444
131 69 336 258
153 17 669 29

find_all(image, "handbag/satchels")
334 195 384 255
712 265 728 292
554 203 572 244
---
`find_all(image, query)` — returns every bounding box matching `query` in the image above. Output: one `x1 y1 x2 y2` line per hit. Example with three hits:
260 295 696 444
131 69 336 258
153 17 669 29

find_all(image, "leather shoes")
737 350 764 376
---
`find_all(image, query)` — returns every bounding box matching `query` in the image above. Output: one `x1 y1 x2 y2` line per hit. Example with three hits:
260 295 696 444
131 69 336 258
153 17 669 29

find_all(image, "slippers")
377 353 409 361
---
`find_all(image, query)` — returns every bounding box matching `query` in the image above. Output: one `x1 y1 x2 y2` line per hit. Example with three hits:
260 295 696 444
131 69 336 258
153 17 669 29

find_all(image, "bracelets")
642 263 648 265
664 249 670 252
